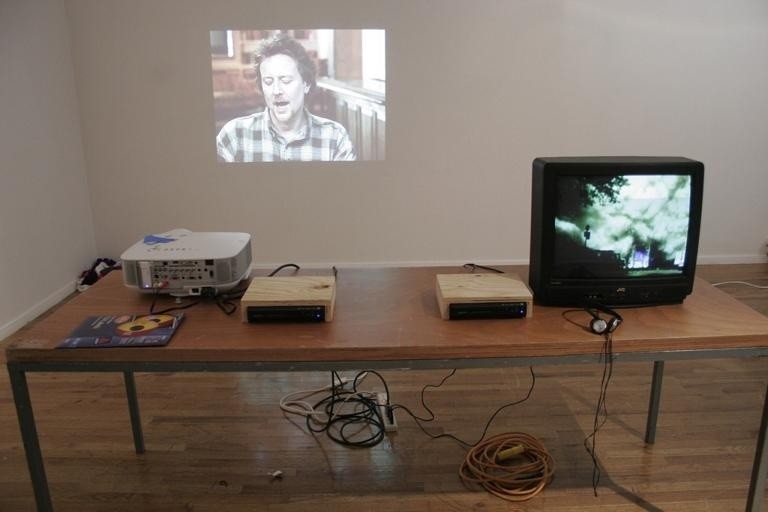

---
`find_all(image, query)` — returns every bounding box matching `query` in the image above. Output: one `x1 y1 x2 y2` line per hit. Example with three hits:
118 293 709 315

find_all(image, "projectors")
120 229 253 297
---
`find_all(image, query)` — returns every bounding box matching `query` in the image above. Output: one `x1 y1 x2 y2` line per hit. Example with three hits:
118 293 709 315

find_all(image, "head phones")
584 304 623 335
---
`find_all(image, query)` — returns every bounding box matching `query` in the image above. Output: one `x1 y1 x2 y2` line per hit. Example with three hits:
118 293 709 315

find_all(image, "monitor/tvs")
529 156 705 311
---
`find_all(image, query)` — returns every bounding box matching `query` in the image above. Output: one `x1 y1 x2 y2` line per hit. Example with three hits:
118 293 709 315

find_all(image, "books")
56 311 183 348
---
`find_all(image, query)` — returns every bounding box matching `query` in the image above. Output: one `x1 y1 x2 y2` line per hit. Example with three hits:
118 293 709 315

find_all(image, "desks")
4 265 767 512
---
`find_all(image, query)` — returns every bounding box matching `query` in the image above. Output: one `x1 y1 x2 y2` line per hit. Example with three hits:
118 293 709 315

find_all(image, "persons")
217 37 356 163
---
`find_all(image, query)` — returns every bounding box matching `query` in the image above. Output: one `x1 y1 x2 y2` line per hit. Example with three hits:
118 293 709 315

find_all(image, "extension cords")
77 261 111 293
